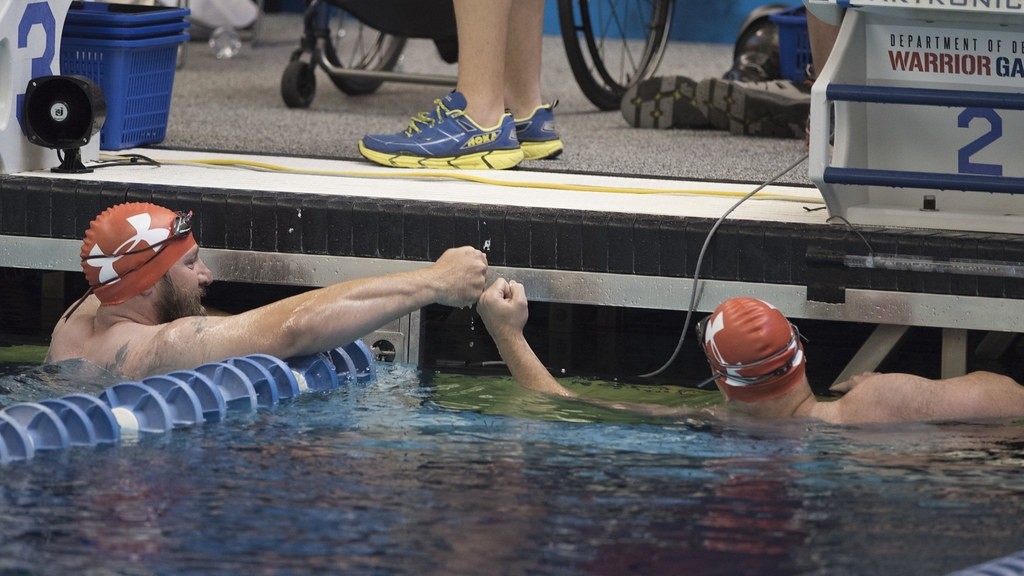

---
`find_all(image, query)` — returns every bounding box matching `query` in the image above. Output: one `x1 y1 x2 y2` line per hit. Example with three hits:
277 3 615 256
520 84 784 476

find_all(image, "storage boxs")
59 0 191 28
61 19 191 39
60 31 190 151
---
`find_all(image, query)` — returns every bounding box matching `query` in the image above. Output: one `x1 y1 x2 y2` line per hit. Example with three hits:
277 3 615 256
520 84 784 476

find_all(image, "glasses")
64 210 191 325
696 315 809 389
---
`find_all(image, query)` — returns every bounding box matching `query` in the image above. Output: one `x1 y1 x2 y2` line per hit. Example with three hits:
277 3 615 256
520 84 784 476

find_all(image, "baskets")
768 6 811 84
61 1 190 150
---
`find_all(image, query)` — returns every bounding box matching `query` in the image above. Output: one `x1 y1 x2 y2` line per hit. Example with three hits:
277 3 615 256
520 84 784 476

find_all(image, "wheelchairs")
282 0 675 117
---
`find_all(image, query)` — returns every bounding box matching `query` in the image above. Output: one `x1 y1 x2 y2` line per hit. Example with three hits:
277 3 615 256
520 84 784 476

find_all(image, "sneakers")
514 100 563 160
358 90 523 170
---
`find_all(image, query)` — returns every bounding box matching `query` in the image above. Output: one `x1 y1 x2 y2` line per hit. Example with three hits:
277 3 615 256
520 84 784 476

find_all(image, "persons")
802 0 846 153
476 278 1024 442
356 0 565 170
45 203 489 376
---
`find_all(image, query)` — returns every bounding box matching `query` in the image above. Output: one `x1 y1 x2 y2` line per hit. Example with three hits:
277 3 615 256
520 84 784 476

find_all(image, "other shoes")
695 76 810 139
620 75 697 129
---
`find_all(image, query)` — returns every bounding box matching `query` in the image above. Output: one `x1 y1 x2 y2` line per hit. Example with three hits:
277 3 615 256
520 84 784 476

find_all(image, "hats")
80 202 196 306
706 298 807 402
